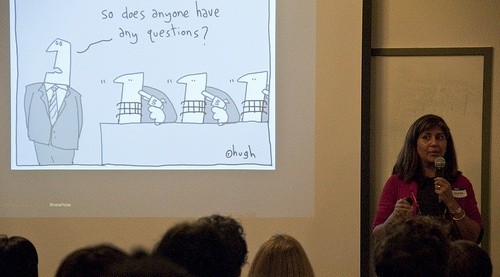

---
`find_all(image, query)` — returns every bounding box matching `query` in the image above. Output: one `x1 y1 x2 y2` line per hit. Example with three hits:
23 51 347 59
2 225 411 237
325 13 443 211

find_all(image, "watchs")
452 205 463 215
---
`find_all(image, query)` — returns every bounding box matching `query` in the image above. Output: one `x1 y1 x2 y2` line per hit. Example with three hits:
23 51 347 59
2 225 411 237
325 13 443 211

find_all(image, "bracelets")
452 211 465 220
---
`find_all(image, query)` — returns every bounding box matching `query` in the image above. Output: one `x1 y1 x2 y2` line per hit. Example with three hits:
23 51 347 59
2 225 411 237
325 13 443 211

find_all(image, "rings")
396 209 400 211
437 185 442 189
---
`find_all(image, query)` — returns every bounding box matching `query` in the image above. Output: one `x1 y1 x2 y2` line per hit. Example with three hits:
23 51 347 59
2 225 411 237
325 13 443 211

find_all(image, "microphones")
434 157 445 202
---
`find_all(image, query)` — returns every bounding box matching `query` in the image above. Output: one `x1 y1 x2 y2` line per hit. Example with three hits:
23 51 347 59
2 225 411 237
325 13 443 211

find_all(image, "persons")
447 239 493 277
0 234 38 277
54 215 249 277
247 234 316 277
371 114 486 245
372 217 451 277
